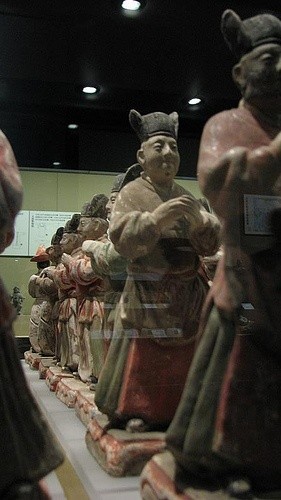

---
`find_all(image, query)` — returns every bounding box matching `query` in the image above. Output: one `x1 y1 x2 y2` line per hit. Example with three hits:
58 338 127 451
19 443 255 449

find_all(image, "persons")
28 109 224 433
9 286 25 315
163 8 281 500
0 132 67 500
